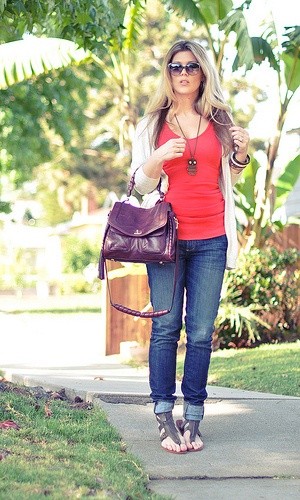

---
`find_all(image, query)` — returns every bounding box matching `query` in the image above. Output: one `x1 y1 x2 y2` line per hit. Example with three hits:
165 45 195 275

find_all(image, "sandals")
155 410 187 454
175 419 204 452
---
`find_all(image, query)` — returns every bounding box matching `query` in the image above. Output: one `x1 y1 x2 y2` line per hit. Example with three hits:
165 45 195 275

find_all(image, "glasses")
167 61 201 76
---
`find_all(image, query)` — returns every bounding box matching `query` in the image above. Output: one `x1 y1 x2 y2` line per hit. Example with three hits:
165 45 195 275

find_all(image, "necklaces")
173 111 202 176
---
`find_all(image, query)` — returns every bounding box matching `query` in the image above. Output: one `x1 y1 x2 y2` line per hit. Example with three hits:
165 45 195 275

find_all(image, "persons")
131 40 250 453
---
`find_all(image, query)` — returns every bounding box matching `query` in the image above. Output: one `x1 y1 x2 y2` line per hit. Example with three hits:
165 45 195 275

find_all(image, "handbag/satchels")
96 166 180 318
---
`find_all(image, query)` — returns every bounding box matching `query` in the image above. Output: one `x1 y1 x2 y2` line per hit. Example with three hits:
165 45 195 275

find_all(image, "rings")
240 137 243 142
